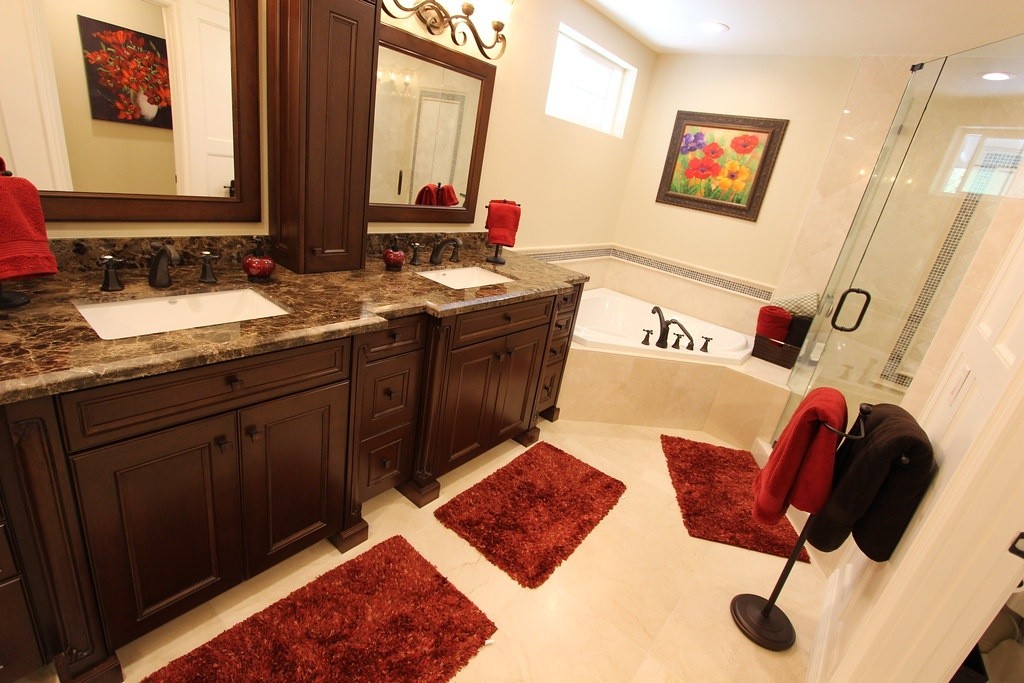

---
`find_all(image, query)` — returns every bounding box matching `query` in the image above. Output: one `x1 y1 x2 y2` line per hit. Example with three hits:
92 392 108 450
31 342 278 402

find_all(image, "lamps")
382 0 513 61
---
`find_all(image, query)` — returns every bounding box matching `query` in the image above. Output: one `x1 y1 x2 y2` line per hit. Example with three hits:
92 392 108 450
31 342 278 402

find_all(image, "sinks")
70 285 291 341
410 264 517 290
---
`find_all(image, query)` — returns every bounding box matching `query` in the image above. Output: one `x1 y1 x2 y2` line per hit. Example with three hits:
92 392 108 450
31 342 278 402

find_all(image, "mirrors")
0 0 264 225
368 21 498 225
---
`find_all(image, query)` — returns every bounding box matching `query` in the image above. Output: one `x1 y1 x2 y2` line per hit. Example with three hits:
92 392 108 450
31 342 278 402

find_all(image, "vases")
136 91 158 119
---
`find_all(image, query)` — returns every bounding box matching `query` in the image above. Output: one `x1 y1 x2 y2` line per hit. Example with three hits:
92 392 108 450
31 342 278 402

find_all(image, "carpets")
143 534 498 683
434 440 627 590
660 434 813 565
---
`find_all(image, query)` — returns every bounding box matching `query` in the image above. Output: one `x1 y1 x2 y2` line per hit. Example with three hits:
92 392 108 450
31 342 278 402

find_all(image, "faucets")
149 241 181 288
651 305 671 349
430 236 463 265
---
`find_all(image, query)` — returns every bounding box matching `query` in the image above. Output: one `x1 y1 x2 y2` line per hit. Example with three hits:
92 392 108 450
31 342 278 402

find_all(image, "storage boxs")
750 333 801 370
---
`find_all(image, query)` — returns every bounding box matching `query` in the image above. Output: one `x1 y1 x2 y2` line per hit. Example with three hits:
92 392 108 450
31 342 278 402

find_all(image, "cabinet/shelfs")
0 285 584 683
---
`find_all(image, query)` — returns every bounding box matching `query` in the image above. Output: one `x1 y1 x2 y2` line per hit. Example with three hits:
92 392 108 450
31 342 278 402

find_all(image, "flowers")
81 28 171 120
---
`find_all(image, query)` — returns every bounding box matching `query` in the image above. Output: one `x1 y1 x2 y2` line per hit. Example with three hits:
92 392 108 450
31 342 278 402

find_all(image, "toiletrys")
382 239 405 272
242 237 276 283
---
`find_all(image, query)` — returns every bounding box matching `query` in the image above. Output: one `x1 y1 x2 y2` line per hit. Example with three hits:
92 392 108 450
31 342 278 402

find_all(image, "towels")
806 403 933 563
751 386 850 527
770 291 821 317
415 184 438 206
0 175 58 280
756 306 792 342
787 315 813 347
484 200 521 247
438 185 459 207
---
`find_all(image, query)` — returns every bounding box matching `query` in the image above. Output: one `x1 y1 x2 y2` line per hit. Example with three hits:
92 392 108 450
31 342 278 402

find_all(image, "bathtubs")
571 286 755 365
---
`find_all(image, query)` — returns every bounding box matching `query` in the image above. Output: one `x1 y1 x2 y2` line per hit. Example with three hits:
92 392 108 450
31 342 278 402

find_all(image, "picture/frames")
655 110 789 224
